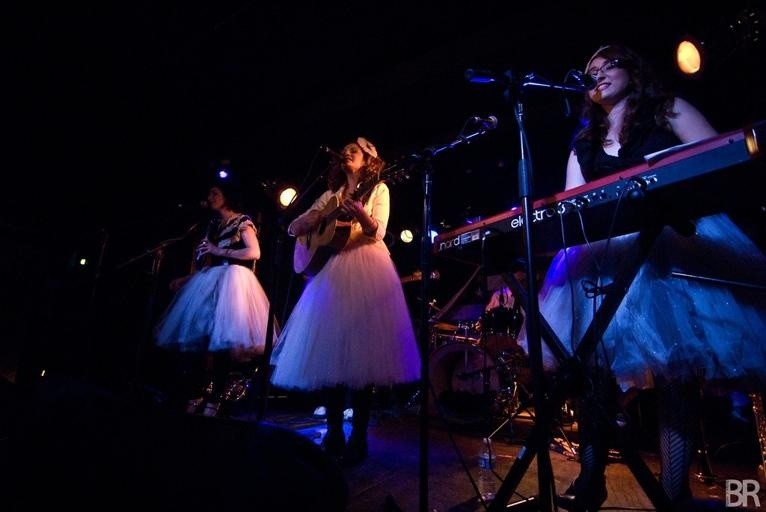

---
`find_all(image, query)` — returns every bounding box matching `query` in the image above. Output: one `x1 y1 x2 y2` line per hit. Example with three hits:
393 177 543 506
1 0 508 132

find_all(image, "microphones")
320 145 344 162
475 115 497 128
571 70 596 90
178 201 208 209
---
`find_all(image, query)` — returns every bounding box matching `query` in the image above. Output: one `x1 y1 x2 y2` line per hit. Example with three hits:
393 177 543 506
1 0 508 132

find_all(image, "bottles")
479 436 498 499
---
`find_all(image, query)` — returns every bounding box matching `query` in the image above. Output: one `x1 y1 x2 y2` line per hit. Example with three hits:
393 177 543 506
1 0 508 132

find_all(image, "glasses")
587 58 618 75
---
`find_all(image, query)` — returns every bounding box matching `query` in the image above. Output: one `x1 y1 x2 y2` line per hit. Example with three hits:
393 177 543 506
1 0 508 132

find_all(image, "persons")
154 180 285 417
262 138 423 461
475 268 531 346
513 41 766 511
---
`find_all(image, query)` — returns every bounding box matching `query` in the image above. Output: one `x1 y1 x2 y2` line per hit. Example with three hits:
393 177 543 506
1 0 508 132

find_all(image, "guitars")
293 164 404 276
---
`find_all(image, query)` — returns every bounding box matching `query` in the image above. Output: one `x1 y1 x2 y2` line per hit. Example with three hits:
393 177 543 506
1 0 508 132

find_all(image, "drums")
481 308 525 340
430 341 513 414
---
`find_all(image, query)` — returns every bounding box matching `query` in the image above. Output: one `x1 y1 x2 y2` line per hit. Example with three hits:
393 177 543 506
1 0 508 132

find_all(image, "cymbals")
435 323 457 330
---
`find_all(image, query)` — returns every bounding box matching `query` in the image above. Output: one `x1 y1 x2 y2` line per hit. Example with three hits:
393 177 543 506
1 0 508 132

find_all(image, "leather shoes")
532 479 608 511
341 434 367 468
321 431 344 460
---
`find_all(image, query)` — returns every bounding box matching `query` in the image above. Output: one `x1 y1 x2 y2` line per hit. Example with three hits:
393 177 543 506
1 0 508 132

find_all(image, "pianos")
434 132 761 274
